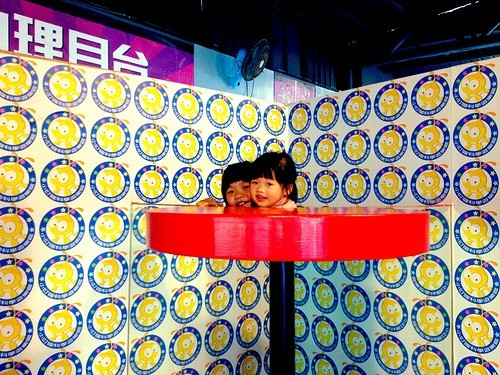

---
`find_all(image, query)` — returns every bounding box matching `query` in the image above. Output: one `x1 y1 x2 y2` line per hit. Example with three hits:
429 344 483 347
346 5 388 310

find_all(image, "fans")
233 39 270 97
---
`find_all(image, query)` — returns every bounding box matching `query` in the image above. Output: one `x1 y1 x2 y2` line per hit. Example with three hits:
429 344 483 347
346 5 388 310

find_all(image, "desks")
144 206 431 375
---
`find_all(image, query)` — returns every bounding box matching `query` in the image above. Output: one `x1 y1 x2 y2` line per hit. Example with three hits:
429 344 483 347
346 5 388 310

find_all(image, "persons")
220 159 253 209
248 151 299 212
